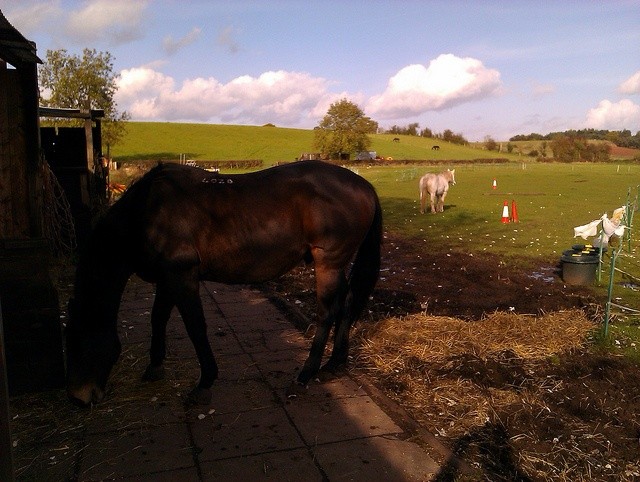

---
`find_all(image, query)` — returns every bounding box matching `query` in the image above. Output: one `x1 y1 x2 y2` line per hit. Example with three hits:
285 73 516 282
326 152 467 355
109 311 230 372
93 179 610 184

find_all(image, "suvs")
560 244 599 287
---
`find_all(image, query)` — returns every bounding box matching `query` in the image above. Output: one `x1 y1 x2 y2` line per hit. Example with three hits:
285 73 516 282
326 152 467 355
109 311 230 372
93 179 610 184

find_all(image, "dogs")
393 138 400 142
64 160 383 410
432 145 440 150
418 168 456 215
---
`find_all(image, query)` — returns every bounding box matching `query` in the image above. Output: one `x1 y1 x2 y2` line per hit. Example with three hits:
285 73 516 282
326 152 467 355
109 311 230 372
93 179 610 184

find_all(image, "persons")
94 155 109 200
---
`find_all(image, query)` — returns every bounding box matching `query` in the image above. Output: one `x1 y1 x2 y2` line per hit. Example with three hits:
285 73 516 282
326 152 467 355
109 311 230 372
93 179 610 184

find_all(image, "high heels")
501 200 510 223
511 199 519 224
493 178 496 189
